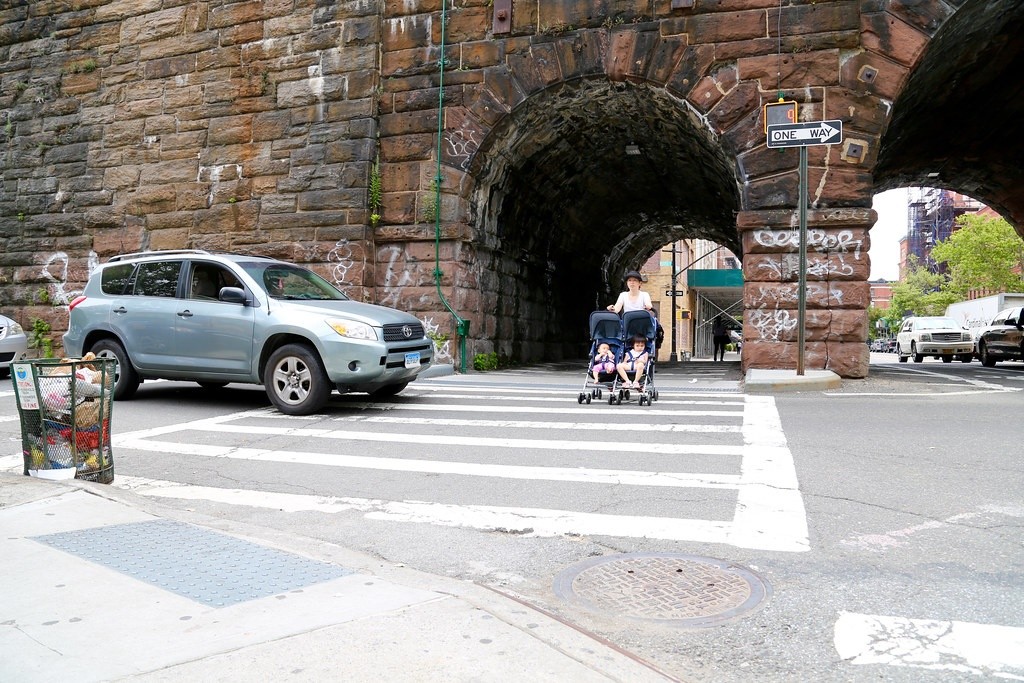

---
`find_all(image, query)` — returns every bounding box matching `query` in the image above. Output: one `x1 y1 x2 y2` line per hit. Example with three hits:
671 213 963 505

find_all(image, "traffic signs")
767 119 843 149
666 290 683 297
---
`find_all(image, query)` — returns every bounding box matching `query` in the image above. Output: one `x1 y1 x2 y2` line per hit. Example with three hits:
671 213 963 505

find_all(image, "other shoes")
633 380 640 389
621 380 632 389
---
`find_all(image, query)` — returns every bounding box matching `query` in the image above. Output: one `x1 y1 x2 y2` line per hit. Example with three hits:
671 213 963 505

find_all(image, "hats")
624 271 642 282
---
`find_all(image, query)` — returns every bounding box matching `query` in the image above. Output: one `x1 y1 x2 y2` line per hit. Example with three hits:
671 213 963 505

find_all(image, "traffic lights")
764 100 798 134
681 310 691 320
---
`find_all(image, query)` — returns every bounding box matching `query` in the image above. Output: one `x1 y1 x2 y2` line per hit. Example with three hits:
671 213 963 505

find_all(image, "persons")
737 340 741 354
712 316 727 362
593 342 615 384
268 278 285 296
616 334 648 388
607 271 653 315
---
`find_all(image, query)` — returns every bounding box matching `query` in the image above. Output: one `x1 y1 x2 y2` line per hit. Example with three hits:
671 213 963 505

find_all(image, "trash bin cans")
10 356 117 485
681 350 691 361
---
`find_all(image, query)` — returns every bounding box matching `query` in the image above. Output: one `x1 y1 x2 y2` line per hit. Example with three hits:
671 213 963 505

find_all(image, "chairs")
193 277 217 299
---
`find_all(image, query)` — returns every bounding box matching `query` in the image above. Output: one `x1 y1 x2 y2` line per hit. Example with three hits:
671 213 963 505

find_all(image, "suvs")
61 248 434 415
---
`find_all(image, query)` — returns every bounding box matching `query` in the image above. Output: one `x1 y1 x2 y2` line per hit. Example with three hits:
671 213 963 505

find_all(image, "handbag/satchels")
724 332 731 344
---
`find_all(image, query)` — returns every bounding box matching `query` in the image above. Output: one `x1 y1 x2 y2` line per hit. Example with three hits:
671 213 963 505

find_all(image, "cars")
897 316 975 363
978 306 1024 368
871 338 897 354
0 315 26 377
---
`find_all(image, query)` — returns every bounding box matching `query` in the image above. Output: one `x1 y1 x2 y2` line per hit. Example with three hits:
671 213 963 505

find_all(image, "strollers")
578 307 659 407
